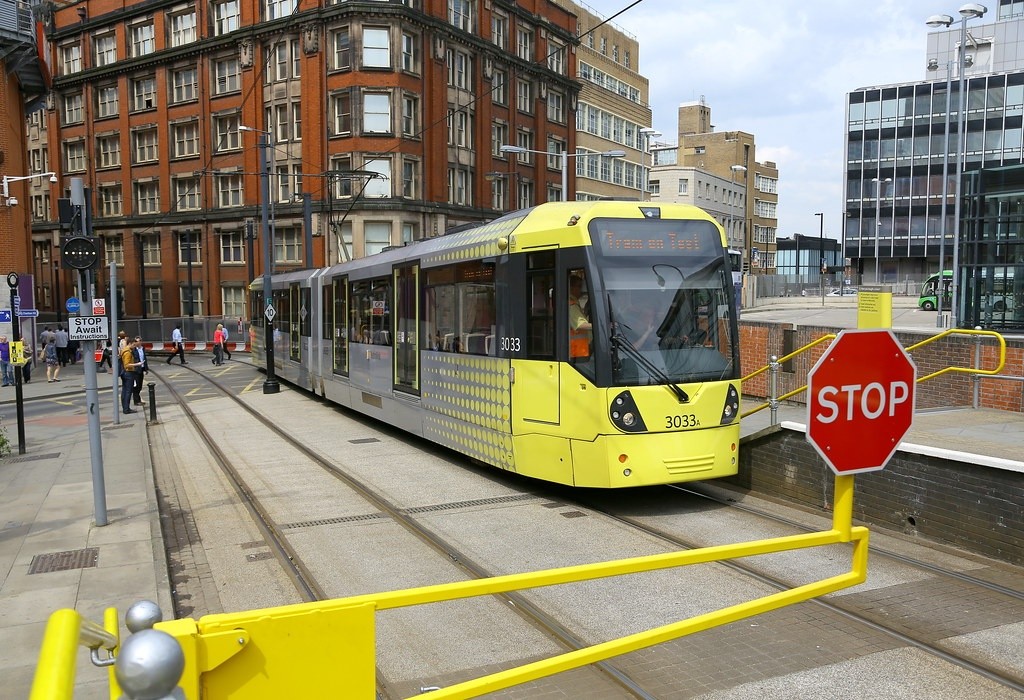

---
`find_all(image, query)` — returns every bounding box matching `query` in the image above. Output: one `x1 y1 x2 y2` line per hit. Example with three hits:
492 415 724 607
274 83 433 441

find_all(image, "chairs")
273 319 495 355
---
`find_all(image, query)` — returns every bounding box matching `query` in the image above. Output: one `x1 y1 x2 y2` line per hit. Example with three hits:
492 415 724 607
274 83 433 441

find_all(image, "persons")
0 334 14 387
98 331 128 374
569 274 688 365
212 324 231 366
117 336 149 414
40 323 81 368
19 336 33 384
779 284 851 297
167 325 189 366
45 337 62 383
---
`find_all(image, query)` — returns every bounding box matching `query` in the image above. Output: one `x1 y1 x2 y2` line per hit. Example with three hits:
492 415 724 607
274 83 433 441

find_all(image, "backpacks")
118 350 129 377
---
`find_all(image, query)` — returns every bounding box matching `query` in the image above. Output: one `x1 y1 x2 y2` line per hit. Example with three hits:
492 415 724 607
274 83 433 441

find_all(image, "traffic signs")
0 296 40 323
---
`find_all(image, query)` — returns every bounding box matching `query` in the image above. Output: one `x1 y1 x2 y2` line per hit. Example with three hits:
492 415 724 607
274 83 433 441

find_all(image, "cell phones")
142 360 145 363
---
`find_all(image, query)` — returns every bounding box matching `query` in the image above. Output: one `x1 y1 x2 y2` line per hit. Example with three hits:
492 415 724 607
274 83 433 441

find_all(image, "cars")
826 289 858 296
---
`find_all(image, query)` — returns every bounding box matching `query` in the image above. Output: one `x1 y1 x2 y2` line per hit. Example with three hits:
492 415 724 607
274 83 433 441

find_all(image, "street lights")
926 3 987 329
639 127 663 200
730 165 748 248
500 145 627 201
238 125 280 394
926 55 975 327
870 178 892 283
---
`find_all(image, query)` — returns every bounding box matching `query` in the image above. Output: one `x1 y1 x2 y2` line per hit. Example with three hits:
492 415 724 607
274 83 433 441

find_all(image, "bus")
917 270 1015 312
696 248 742 320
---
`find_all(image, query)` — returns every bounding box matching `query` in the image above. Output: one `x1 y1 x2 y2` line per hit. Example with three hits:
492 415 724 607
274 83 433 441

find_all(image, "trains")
245 201 741 488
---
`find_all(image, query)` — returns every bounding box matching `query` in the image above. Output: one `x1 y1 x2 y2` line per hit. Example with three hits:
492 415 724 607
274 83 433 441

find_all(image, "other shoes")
212 360 215 364
216 364 221 365
166 359 171 365
181 362 188 364
1 383 9 387
228 353 231 360
123 408 137 414
220 361 226 364
134 402 145 406
54 379 60 382
47 381 54 383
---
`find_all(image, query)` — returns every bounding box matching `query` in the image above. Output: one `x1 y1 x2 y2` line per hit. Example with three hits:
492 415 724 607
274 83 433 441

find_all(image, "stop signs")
806 328 917 477
67 315 109 341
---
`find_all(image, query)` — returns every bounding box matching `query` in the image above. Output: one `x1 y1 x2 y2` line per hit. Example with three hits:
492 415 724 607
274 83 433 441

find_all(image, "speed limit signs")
263 303 277 323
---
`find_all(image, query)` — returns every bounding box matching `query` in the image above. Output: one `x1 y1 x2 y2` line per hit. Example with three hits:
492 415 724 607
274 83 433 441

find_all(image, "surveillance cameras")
49 177 57 184
701 164 704 168
877 223 881 226
9 199 18 207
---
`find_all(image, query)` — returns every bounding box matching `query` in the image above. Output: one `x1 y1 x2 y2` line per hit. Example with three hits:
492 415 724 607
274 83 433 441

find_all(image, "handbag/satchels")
39 344 48 362
